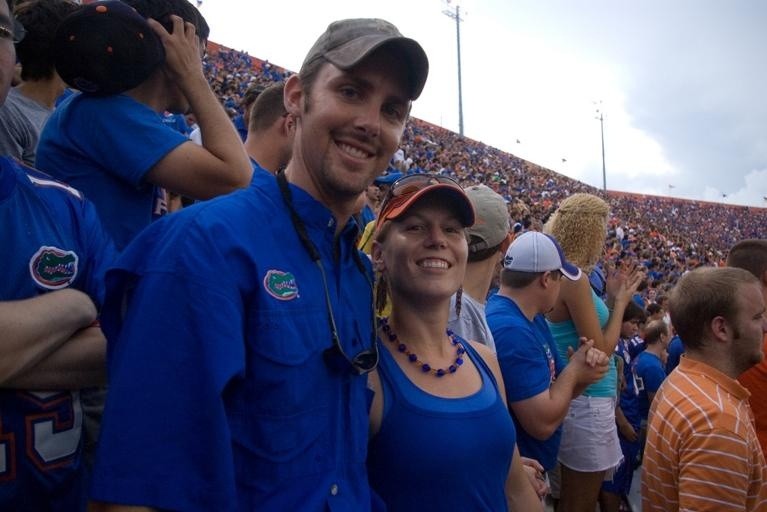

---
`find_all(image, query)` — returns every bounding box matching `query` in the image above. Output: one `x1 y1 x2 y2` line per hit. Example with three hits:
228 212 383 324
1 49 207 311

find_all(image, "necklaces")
379 318 466 377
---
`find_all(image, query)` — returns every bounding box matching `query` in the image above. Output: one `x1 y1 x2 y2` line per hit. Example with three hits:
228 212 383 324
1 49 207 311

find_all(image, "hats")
460 184 511 252
503 230 582 281
50 2 167 95
301 16 430 100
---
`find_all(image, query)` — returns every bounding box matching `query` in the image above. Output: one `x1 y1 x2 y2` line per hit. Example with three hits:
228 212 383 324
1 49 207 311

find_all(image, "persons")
364 175 546 512
0 0 118 512
597 306 645 512
38 0 252 252
225 86 268 142
91 18 428 512
727 240 767 459
243 82 298 171
487 231 610 472
630 320 674 407
541 193 644 512
643 267 767 512
448 183 512 356
403 118 461 179
3 1 94 171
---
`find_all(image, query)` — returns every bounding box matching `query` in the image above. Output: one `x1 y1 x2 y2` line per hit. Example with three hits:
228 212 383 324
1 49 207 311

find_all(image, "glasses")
299 235 381 375
0 15 26 43
